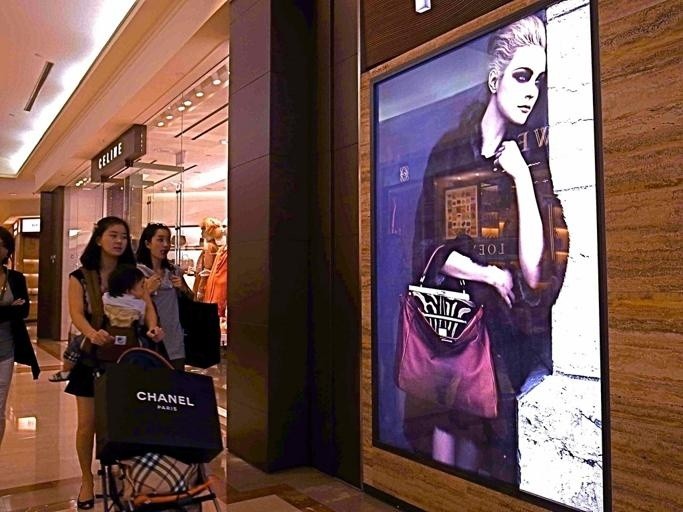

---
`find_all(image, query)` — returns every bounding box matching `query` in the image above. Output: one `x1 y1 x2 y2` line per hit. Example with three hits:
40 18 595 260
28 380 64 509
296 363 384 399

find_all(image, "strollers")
89 328 221 512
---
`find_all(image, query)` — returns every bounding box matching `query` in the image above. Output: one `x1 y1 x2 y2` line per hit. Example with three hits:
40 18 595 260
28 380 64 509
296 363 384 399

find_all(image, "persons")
194 218 224 306
49 267 146 382
0 226 41 444
205 218 228 320
65 217 165 508
404 18 572 489
136 220 194 371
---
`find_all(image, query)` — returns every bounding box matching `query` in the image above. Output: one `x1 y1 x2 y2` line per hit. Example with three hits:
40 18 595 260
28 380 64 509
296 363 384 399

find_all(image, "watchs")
182 287 191 296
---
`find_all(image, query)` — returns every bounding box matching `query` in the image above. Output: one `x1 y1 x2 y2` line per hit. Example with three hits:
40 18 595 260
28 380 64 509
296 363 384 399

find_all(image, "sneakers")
49 371 70 382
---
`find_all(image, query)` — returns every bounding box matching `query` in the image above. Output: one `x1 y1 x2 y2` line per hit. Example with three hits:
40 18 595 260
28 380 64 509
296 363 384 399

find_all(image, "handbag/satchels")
94 363 223 463
118 453 199 500
180 297 221 368
394 248 498 419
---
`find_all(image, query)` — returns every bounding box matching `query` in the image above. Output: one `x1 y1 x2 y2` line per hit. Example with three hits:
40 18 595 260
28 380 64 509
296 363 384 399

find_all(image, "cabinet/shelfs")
164 225 228 275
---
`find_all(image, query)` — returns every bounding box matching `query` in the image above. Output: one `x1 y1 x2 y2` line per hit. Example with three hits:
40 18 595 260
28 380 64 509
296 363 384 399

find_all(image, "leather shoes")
77 486 95 509
